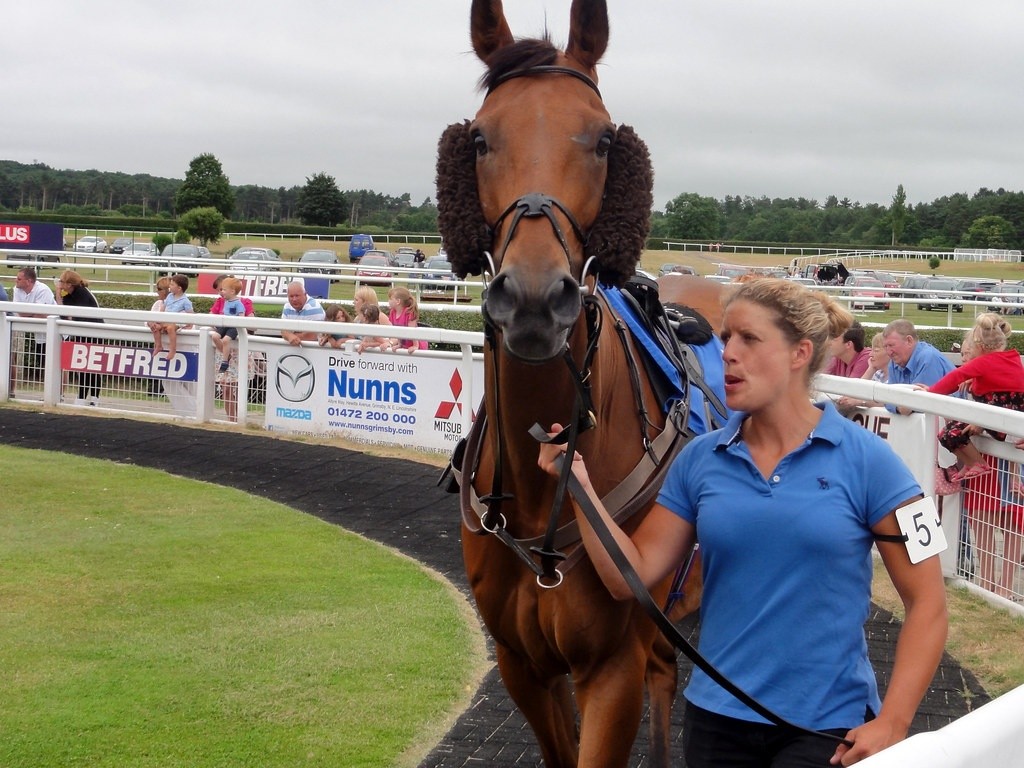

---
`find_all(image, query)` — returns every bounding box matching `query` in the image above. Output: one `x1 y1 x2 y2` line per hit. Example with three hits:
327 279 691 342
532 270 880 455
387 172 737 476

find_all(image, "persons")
144 273 194 398
208 274 257 422
538 278 951 768
13 268 70 397
822 313 1024 599
281 281 326 347
56 271 105 405
318 286 427 355
0 283 17 398
414 249 421 268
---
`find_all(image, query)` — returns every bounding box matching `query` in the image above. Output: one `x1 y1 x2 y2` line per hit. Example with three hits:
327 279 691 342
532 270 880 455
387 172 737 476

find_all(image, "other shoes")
90 396 98 407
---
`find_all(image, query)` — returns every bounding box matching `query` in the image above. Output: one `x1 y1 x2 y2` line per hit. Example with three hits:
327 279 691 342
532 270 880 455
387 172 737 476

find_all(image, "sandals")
951 464 992 482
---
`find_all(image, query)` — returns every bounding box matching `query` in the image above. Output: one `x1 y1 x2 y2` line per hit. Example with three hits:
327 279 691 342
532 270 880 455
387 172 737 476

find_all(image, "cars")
7 235 1024 314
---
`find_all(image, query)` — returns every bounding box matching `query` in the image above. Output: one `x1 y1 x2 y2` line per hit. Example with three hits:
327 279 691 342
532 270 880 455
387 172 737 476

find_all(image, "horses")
460 1 734 768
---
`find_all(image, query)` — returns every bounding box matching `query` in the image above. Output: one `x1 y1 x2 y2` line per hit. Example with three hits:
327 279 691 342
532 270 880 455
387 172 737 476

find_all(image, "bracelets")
862 400 867 406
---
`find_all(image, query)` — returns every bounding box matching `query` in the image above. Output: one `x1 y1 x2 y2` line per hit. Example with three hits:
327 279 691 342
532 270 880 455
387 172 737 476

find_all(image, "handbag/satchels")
937 467 962 495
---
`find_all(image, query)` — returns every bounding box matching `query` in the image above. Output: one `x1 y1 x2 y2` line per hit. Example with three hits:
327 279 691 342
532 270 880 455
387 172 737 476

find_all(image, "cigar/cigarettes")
299 344 302 348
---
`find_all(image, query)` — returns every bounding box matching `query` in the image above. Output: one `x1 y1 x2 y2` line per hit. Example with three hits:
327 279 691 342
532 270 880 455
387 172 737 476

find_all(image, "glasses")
157 287 164 291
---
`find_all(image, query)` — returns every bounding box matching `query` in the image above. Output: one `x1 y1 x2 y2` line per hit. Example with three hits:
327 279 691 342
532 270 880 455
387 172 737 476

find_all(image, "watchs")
388 340 394 347
